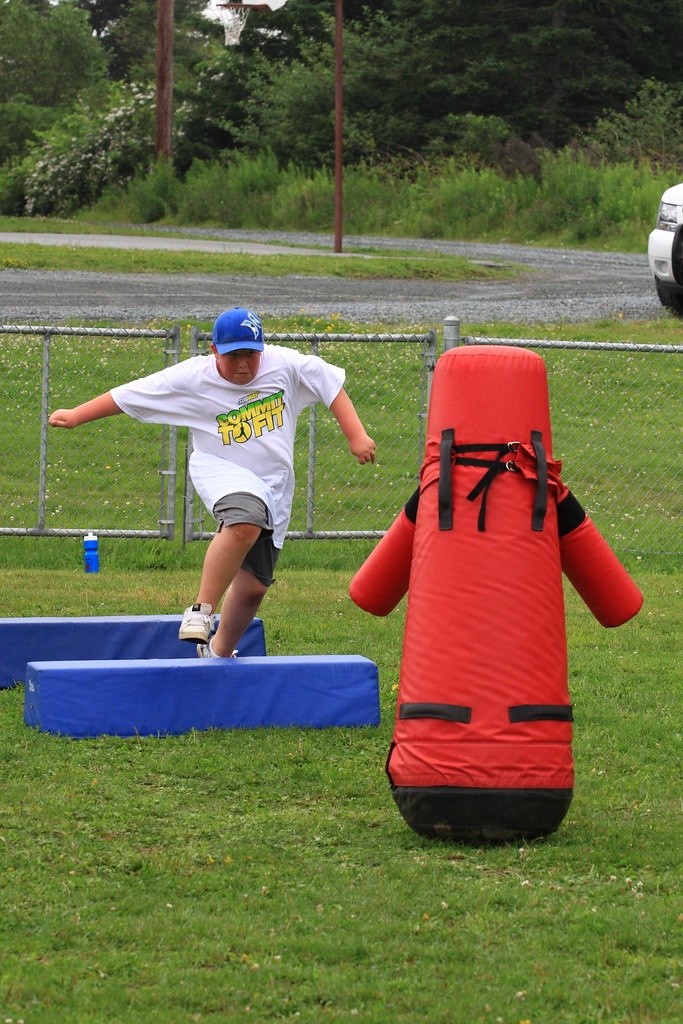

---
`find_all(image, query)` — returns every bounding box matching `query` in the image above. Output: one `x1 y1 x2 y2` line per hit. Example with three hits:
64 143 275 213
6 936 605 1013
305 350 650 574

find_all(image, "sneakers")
196 635 237 658
178 602 213 645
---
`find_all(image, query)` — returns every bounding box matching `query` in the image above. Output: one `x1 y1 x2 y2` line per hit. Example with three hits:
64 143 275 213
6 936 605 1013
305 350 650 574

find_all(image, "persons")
48 307 377 665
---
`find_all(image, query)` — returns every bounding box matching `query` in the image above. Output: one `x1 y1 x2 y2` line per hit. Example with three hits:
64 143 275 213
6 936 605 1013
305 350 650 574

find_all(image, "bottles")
84 531 99 575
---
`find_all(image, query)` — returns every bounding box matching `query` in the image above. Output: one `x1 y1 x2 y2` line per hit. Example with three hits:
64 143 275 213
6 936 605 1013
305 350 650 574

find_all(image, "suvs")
648 181 682 309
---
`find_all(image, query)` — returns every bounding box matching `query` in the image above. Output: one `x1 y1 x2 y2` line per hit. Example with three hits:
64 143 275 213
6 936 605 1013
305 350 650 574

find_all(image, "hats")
212 305 265 355
